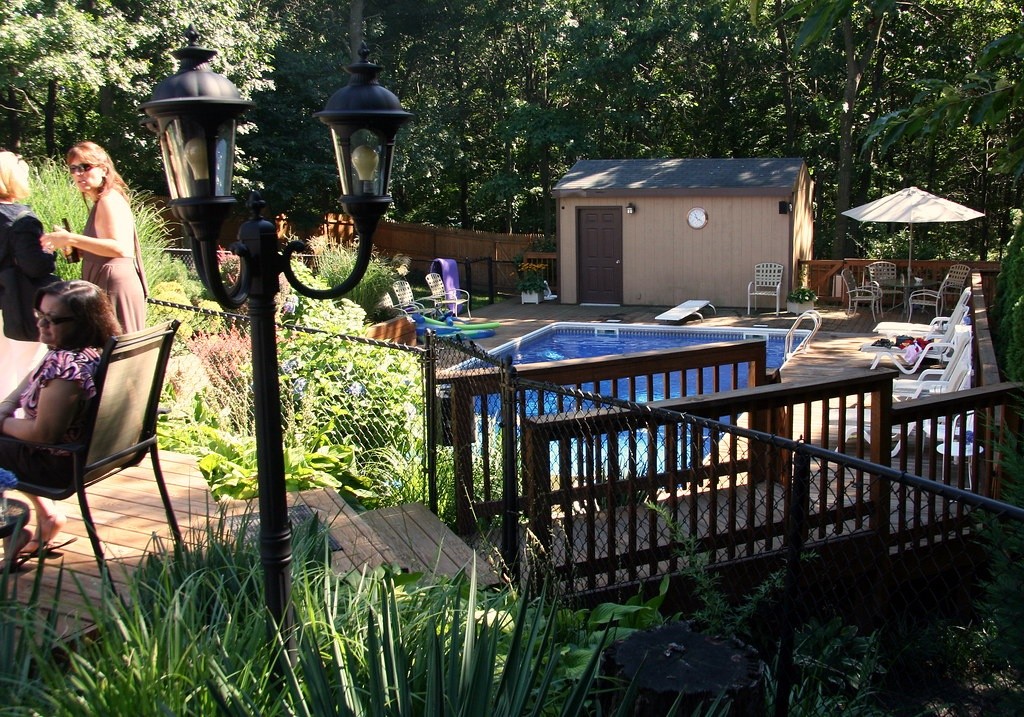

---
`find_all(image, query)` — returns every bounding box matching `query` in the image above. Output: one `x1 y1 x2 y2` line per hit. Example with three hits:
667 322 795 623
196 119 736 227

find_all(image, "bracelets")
2 400 17 405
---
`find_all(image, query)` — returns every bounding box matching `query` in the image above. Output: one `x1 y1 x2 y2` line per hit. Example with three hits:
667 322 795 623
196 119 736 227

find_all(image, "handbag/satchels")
0 210 63 341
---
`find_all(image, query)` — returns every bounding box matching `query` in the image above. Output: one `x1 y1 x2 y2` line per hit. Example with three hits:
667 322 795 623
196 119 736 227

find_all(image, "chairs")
377 281 443 320
866 261 907 314
907 273 950 323
425 273 472 320
835 287 974 458
0 319 188 600
841 268 884 322
944 264 970 310
748 263 784 316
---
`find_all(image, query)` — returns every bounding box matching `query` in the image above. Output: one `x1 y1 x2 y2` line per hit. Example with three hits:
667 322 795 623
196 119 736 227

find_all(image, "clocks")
686 207 708 230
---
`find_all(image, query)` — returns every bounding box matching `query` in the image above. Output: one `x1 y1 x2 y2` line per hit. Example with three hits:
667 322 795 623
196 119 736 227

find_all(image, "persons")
0 151 60 342
40 142 149 335
0 279 123 573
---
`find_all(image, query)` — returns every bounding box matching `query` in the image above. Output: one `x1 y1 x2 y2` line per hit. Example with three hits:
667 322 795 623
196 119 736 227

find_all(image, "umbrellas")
840 186 986 281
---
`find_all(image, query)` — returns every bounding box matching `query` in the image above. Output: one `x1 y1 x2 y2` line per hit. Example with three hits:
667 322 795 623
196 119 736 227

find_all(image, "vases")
0 495 8 521
787 301 814 316
521 290 544 304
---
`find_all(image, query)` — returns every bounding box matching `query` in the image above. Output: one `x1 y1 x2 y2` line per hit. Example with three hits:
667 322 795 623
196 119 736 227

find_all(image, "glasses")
70 163 101 175
32 307 81 326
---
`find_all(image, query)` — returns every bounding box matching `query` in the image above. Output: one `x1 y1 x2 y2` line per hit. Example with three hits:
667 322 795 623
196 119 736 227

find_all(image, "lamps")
312 41 415 235
625 202 635 214
135 22 253 243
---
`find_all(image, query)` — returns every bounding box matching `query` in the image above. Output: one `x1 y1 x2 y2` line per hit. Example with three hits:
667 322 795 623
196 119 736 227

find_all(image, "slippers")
21 537 79 556
0 544 47 572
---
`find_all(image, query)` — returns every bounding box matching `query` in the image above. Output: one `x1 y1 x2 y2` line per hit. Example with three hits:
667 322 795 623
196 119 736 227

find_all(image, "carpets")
227 504 344 555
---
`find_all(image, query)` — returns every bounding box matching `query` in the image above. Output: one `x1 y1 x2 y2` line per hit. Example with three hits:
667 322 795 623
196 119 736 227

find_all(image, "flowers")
509 263 548 294
785 288 818 304
0 468 20 510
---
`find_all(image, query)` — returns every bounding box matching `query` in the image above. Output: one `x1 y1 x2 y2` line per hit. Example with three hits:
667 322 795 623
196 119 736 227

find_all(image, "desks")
937 441 984 491
0 498 30 539
880 278 938 319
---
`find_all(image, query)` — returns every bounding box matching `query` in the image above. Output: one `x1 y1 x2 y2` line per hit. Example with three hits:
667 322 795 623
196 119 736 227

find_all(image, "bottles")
446 316 453 326
412 314 425 324
62 218 80 264
